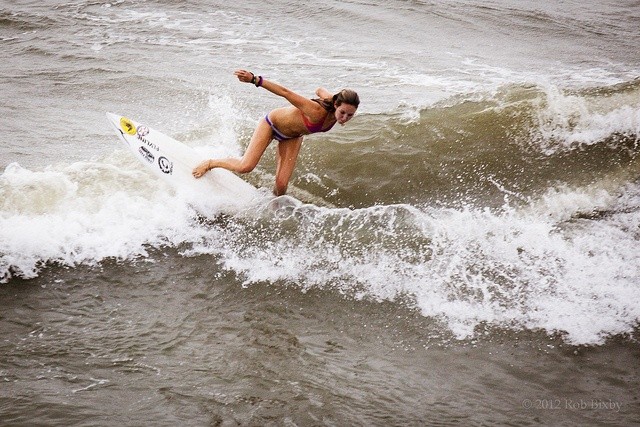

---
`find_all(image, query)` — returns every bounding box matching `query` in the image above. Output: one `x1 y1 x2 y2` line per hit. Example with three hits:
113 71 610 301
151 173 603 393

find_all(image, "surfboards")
106 112 266 211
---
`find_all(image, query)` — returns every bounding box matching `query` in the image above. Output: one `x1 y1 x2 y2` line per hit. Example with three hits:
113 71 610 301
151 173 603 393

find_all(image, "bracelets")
256 76 263 86
250 72 256 84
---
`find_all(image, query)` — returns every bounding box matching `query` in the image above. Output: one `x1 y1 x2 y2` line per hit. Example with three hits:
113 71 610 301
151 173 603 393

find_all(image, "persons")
193 70 360 212
